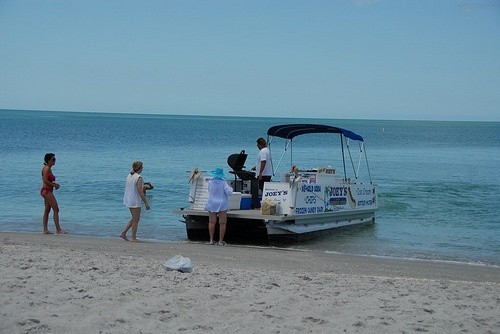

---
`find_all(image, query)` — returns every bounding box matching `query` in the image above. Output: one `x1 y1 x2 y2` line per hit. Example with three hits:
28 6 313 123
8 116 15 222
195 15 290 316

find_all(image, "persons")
251 138 274 210
206 168 233 246
118 161 151 242
40 152 68 235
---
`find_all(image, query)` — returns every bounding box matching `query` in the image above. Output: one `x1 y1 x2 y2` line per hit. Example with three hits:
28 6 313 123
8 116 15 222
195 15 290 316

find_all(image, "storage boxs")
229 192 252 210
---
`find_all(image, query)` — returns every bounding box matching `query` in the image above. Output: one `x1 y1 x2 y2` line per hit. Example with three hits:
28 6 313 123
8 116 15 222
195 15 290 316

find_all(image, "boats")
171 124 379 246
